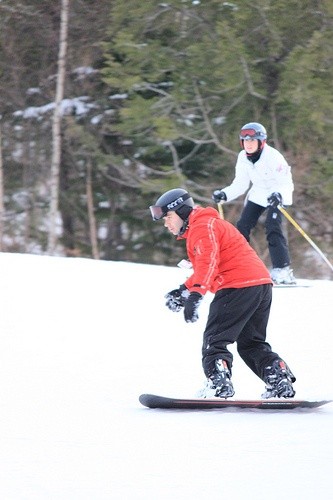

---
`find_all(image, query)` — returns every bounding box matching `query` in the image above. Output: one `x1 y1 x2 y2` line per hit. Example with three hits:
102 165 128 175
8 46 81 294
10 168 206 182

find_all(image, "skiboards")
187 276 311 288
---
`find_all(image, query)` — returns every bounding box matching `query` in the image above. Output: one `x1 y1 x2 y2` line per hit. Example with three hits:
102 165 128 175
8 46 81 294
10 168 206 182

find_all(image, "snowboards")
139 394 333 409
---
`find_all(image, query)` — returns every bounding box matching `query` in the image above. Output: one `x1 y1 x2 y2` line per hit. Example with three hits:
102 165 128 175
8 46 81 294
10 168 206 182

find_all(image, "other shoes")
270 267 297 285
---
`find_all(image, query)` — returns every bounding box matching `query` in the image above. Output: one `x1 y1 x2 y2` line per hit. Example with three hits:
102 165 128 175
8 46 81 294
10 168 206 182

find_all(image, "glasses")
241 128 259 138
150 203 167 221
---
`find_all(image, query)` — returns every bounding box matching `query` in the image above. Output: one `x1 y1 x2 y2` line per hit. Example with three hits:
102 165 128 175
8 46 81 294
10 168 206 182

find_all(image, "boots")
261 359 298 399
199 359 236 399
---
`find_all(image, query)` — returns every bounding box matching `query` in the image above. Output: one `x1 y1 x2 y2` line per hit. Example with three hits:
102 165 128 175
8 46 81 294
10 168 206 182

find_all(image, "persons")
213 123 295 284
149 189 296 399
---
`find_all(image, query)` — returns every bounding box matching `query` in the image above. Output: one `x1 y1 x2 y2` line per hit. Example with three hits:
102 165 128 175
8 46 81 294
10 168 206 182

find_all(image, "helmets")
149 189 195 236
240 122 267 157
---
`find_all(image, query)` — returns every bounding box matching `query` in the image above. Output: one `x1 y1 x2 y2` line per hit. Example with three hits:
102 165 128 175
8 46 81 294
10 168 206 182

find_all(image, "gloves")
164 284 186 312
267 192 283 209
212 190 227 203
183 291 203 322
